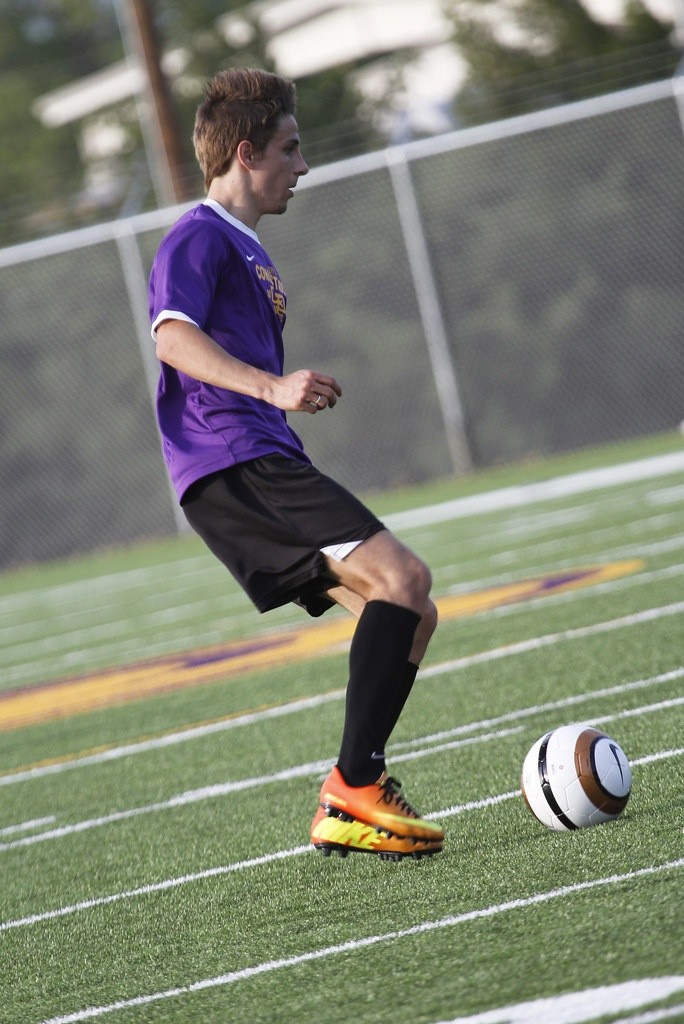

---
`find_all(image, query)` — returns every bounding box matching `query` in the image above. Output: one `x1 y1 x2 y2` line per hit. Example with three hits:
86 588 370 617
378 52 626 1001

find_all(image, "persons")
147 66 448 862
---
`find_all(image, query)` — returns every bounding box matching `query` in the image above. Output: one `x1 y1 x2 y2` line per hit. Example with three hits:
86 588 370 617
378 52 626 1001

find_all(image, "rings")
314 395 321 404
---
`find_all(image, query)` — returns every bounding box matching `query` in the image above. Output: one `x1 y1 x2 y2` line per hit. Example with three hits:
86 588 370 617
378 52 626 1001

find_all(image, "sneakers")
308 803 443 861
319 765 445 845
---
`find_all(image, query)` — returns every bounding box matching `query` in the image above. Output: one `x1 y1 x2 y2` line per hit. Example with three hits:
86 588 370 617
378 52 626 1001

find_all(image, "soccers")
520 724 632 832
